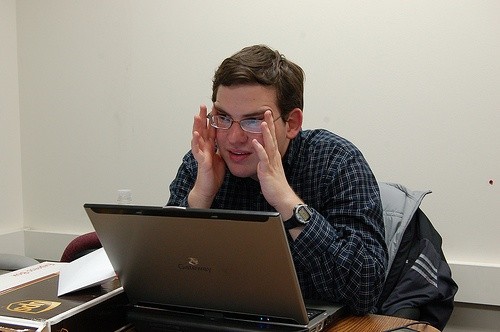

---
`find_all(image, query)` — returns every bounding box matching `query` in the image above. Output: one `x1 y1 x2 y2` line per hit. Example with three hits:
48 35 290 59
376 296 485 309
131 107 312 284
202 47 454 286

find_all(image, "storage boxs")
0 261 132 332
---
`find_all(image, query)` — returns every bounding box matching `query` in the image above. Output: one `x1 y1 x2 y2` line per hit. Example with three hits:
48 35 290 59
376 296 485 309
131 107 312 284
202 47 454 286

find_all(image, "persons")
165 44 389 316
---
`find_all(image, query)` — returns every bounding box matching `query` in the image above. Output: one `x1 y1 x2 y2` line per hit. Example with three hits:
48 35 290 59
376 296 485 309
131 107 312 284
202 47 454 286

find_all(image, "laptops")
84 203 347 332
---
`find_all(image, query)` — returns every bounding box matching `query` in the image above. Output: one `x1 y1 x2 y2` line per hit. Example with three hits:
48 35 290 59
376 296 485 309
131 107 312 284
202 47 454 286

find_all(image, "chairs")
377 182 458 330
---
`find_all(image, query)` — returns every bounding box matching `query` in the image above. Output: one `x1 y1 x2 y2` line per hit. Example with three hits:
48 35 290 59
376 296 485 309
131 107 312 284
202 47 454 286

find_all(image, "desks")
323 311 442 332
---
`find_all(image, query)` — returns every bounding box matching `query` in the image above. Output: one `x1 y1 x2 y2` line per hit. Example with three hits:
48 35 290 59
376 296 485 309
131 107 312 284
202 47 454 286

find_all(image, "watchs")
283 203 313 229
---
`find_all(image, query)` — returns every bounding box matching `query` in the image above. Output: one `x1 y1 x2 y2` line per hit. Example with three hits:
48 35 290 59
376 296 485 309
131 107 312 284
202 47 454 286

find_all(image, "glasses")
205 111 281 134
